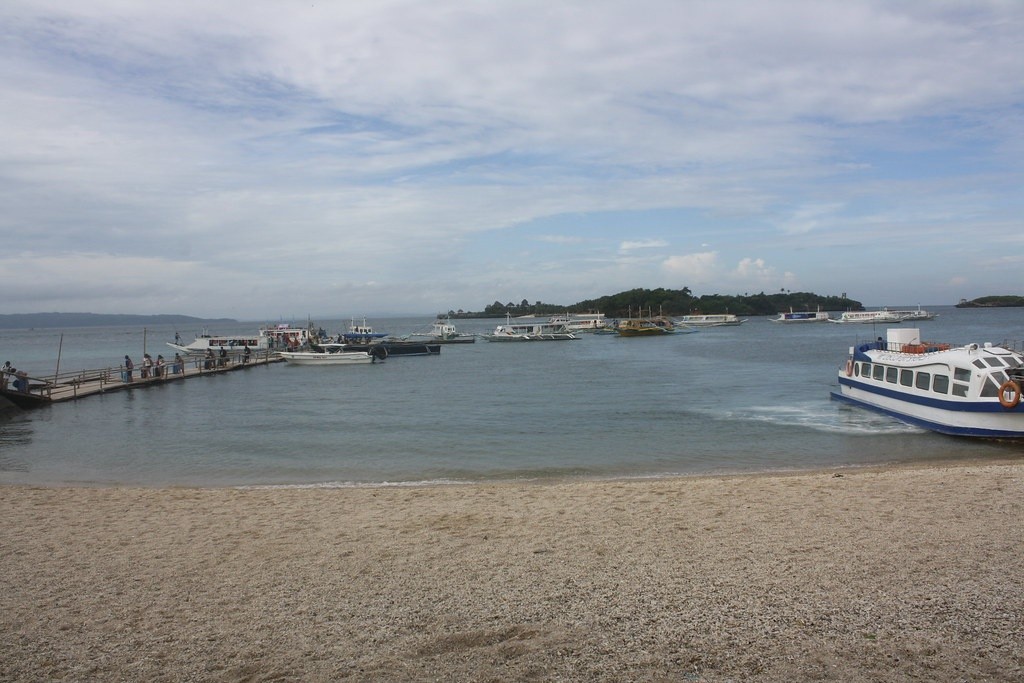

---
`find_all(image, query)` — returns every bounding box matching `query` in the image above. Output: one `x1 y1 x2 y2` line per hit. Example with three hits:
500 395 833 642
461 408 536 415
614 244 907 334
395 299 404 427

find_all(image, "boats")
827 309 902 323
165 334 268 357
767 311 831 322
827 327 1024 440
477 307 582 339
544 315 607 330
613 319 672 335
862 313 902 324
680 314 749 326
595 303 699 333
903 303 941 320
258 314 477 366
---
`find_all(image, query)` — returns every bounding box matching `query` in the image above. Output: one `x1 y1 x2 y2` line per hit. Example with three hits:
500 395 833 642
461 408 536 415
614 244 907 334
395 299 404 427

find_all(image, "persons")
205 348 216 368
1 360 12 389
269 333 348 351
143 354 165 378
220 347 226 367
175 353 182 373
125 355 133 382
11 368 30 393
175 333 180 345
244 345 250 362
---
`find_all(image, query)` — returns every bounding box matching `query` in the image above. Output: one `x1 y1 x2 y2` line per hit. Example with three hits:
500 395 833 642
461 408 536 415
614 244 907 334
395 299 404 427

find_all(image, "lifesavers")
998 381 1020 408
846 360 853 377
